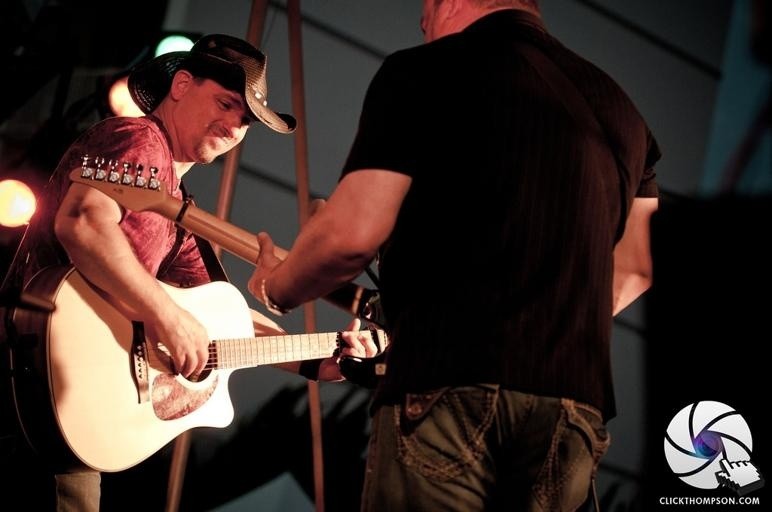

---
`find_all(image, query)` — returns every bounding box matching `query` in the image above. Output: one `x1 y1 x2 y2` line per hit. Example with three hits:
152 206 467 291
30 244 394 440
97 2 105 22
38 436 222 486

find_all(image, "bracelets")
298 357 327 382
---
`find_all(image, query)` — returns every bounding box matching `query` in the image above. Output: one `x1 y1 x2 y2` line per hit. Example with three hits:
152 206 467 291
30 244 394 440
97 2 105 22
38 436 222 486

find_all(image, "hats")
126 33 299 136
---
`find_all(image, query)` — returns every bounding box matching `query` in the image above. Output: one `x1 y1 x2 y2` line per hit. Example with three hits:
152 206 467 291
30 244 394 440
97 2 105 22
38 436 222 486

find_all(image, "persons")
238 0 666 511
0 29 393 511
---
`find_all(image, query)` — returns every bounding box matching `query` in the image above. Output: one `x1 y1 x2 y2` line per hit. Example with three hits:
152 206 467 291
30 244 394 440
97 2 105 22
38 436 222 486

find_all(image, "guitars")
12 262 389 473
69 155 390 395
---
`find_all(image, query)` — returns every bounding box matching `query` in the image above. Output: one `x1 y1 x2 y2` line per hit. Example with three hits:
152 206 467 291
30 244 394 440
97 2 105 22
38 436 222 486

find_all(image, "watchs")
260 282 293 317
